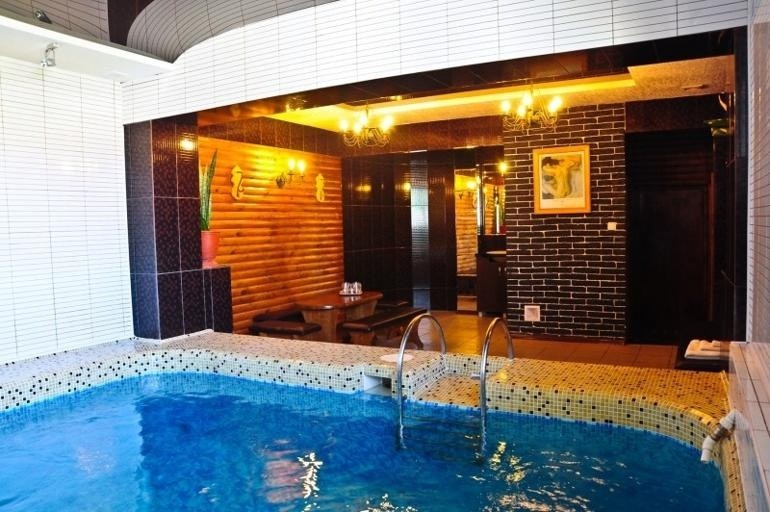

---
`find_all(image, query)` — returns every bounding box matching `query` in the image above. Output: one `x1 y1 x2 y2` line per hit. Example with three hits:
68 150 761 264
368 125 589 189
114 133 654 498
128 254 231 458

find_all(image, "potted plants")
199 148 219 267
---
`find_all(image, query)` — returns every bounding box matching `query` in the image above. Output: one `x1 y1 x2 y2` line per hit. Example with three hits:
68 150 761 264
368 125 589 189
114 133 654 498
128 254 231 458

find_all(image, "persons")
541 157 577 199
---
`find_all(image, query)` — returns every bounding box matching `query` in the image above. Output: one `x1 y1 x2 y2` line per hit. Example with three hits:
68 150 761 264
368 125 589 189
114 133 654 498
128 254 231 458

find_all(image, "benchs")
250 283 425 349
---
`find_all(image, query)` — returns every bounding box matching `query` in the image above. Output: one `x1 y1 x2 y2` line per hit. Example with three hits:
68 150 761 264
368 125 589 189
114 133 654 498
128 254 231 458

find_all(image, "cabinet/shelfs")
474 234 507 318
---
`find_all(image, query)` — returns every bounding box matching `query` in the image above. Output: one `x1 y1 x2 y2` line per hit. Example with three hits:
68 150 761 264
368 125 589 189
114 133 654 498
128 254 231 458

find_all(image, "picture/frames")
531 144 591 216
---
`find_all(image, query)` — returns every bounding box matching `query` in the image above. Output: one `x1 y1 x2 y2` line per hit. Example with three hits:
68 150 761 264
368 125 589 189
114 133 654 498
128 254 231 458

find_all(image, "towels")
685 335 722 360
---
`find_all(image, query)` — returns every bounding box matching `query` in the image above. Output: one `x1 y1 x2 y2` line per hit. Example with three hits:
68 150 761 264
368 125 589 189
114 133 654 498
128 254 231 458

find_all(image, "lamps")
286 159 305 182
494 71 561 133
339 103 399 149
